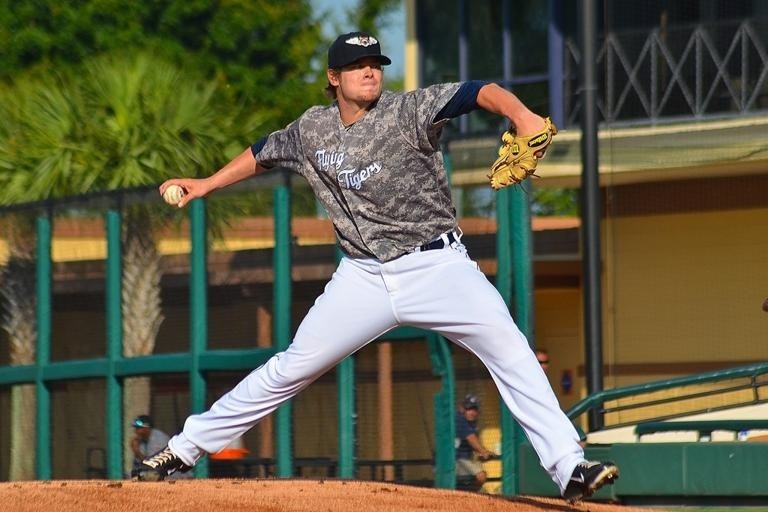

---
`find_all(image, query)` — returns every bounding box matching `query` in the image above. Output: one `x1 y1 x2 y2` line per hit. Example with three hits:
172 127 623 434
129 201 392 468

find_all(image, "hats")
130 414 151 428
328 32 391 68
462 394 480 411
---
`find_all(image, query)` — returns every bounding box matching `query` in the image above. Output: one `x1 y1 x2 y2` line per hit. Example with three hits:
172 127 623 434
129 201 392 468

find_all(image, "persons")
131 31 619 505
537 349 549 373
131 413 192 480
455 396 494 494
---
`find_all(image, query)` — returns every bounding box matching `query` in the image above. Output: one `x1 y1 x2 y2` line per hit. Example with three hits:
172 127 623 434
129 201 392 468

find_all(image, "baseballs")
162 185 185 206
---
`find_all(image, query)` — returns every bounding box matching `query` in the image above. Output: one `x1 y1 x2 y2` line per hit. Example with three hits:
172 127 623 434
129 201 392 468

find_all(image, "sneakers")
131 444 192 481
565 460 620 505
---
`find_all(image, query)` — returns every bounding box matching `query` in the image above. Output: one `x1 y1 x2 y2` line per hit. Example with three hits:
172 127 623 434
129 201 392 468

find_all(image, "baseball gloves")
487 119 557 191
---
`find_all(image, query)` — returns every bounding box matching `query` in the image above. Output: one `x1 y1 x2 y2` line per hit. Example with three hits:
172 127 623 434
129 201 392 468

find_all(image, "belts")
404 227 461 256
